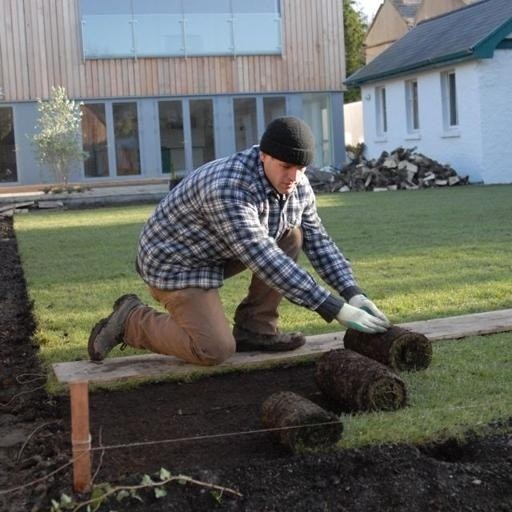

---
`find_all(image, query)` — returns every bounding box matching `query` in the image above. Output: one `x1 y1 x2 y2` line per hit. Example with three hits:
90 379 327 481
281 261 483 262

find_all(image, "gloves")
336 294 390 334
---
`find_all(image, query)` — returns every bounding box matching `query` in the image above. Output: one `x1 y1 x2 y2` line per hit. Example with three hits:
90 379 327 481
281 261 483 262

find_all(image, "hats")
259 117 315 166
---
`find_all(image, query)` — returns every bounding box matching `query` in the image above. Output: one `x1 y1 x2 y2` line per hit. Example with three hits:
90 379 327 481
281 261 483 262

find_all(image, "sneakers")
232 324 305 352
88 294 144 360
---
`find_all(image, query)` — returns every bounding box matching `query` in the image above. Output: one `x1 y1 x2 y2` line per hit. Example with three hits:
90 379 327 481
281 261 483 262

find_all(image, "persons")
88 115 393 367
